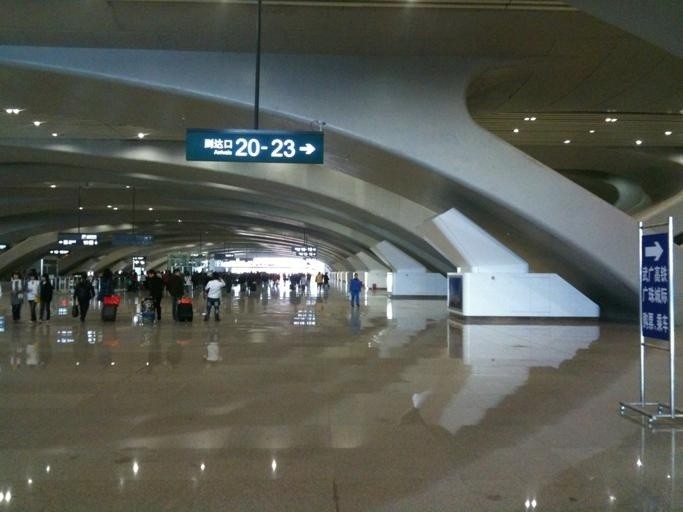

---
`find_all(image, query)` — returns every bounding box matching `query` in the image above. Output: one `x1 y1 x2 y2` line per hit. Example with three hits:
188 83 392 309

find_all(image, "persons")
350 273 362 307
9 324 226 372
222 270 331 292
9 268 225 323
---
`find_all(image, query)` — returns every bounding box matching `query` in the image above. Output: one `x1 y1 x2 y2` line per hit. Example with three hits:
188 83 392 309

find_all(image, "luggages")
140 296 156 322
101 294 120 320
173 298 193 322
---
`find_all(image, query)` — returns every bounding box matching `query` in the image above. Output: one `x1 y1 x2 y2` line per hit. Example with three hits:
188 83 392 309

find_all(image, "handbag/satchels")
33 294 40 303
88 285 96 297
72 296 78 318
